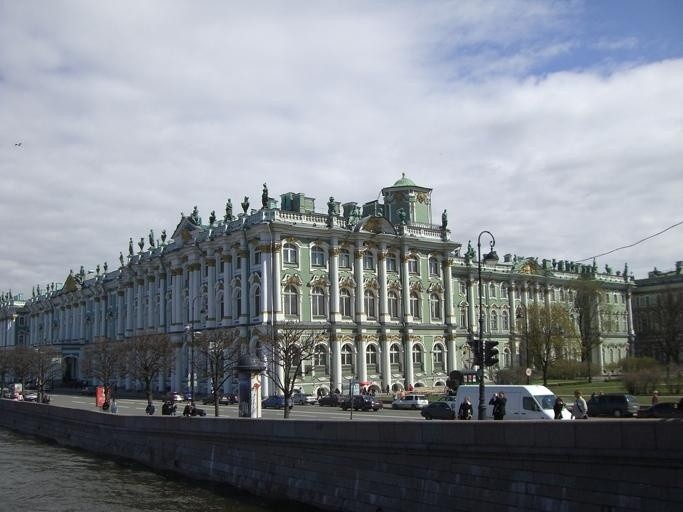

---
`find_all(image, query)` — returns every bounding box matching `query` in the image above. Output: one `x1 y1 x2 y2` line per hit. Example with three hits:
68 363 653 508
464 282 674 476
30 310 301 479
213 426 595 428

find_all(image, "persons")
111 398 118 413
299 386 304 394
208 210 215 225
399 207 406 222
552 397 564 419
118 228 167 266
182 400 192 416
358 387 376 397
260 183 268 208
441 209 448 229
315 388 322 399
570 389 588 419
172 391 192 403
144 400 154 416
102 399 110 411
42 390 49 403
171 399 178 416
326 196 340 216
488 391 507 419
400 390 404 399
392 391 397 400
240 196 249 213
651 390 659 405
161 400 170 416
190 205 200 225
456 394 473 420
17 392 23 400
466 239 471 253
221 392 237 405
223 198 232 223
334 387 340 394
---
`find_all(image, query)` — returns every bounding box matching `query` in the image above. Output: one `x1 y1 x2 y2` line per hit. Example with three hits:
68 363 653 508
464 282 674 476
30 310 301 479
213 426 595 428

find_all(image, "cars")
203 392 240 406
421 396 456 421
292 392 317 406
318 393 345 406
166 389 192 405
637 401 680 417
340 394 383 412
588 394 639 417
1 382 50 402
261 394 293 409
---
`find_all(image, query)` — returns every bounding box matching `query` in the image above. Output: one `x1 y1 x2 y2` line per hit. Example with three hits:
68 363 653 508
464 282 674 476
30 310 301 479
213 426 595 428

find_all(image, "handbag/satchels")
584 414 587 419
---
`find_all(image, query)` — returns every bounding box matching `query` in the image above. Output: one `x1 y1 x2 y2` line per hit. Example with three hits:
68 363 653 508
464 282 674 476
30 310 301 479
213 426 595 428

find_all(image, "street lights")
516 303 530 384
189 295 206 402
475 232 499 420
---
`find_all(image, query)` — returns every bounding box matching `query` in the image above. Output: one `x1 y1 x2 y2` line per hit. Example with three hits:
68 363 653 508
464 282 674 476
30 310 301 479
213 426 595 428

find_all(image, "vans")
391 394 428 409
456 384 575 422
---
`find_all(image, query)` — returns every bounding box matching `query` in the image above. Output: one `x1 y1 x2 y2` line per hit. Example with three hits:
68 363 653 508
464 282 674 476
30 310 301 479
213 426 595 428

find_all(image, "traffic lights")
484 340 500 366
468 341 481 369
